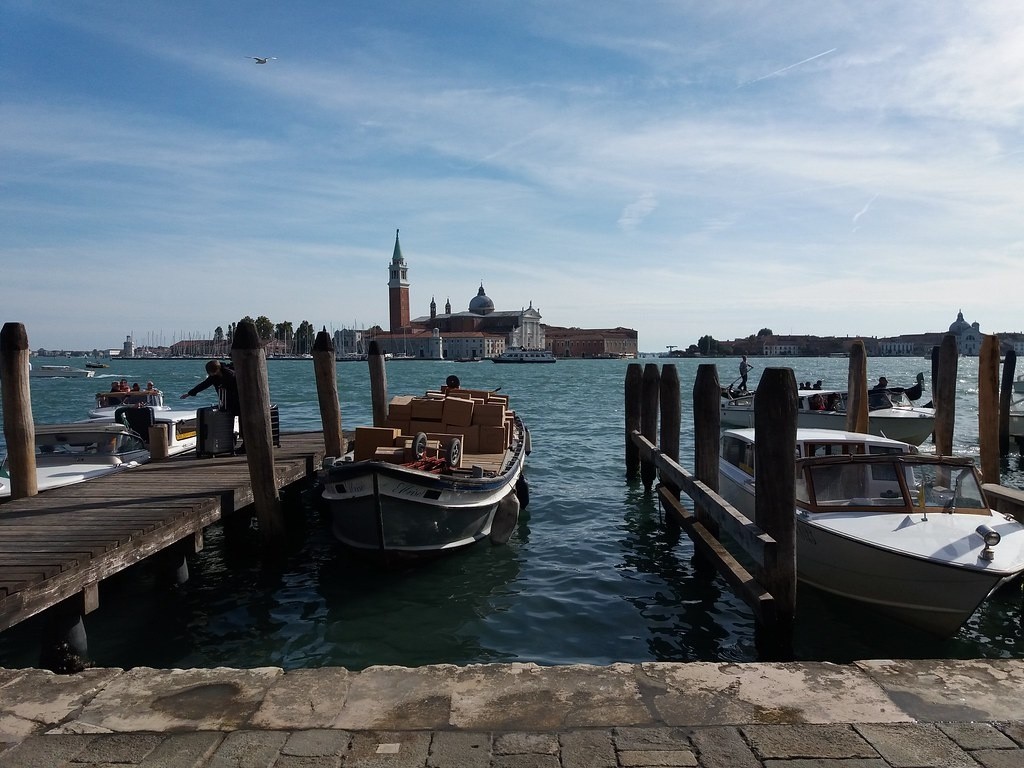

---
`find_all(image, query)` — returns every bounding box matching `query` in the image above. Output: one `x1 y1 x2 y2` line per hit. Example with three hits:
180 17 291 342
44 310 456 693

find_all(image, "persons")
737 355 754 392
104 378 161 406
798 377 888 410
179 352 247 455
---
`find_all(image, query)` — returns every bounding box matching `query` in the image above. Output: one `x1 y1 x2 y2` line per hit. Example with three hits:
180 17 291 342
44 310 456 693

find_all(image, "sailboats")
124 322 414 362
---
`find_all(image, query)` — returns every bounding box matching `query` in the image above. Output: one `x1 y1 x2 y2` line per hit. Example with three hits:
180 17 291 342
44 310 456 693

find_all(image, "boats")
0 389 244 500
491 346 557 363
611 353 634 359
1014 380 1024 394
976 409 1024 438
29 363 96 378
317 375 533 555
85 360 110 368
718 382 936 449
716 426 1024 634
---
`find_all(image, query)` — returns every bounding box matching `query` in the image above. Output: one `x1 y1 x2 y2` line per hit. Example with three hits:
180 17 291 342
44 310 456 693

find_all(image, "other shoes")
236 442 246 454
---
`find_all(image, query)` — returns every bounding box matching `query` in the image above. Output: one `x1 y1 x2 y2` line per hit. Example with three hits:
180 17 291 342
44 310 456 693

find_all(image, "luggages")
196 386 235 458
269 403 281 448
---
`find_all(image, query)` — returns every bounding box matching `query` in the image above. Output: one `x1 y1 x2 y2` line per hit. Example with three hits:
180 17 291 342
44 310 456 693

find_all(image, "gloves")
180 394 189 399
217 384 226 390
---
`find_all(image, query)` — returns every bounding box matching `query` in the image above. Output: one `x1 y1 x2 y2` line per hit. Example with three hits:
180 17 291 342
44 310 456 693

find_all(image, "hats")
147 381 154 386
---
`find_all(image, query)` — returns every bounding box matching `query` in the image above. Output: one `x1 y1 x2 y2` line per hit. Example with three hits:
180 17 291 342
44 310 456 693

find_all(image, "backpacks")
219 361 236 382
